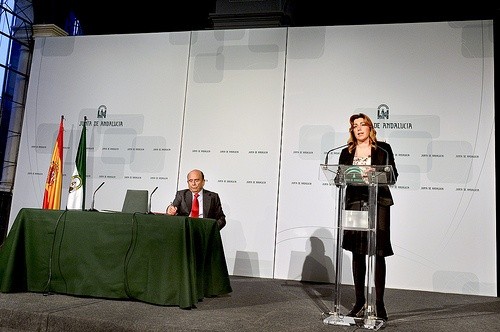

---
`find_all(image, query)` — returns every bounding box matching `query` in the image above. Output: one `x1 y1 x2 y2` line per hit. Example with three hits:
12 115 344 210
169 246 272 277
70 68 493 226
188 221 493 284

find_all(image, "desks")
0 207 233 309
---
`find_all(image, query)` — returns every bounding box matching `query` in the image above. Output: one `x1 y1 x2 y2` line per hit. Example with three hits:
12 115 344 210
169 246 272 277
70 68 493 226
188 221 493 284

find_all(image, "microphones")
372 142 389 165
148 187 158 214
325 142 354 165
89 182 105 212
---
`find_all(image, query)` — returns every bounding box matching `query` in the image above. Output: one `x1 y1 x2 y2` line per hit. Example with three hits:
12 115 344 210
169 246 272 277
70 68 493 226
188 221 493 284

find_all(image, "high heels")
347 302 365 317
374 304 388 321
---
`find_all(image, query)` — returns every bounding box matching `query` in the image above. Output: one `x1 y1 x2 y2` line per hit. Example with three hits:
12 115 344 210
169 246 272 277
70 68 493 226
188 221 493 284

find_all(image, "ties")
191 193 200 218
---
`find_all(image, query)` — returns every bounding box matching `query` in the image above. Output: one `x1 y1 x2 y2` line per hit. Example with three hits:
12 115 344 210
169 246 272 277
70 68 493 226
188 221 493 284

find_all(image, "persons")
166 169 226 231
334 113 399 322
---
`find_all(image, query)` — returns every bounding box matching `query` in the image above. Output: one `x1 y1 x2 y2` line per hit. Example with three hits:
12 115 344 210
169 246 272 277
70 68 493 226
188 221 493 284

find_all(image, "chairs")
122 189 148 214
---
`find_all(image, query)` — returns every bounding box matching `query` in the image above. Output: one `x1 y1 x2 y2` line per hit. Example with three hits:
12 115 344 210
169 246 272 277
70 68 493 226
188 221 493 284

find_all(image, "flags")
42 115 64 211
66 116 87 210
